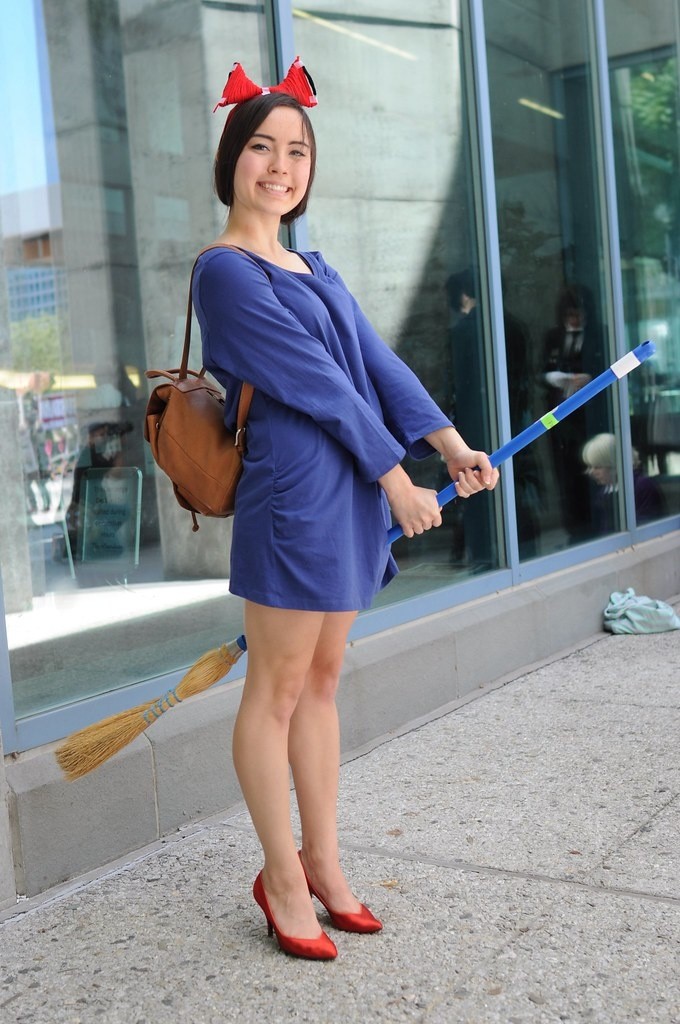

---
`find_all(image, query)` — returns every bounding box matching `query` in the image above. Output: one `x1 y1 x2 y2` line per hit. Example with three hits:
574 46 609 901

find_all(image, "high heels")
252 873 337 960
299 849 383 935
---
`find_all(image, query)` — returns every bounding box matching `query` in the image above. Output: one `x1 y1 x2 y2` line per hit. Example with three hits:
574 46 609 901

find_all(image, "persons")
581 433 671 534
190 89 500 960
56 420 133 560
534 284 611 546
443 270 543 575
17 367 54 514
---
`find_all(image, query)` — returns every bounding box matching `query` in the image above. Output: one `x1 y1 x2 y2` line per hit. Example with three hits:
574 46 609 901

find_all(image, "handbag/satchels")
148 366 248 518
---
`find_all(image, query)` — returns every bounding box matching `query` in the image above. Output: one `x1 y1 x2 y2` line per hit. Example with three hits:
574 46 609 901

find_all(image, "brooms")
54 336 655 785
